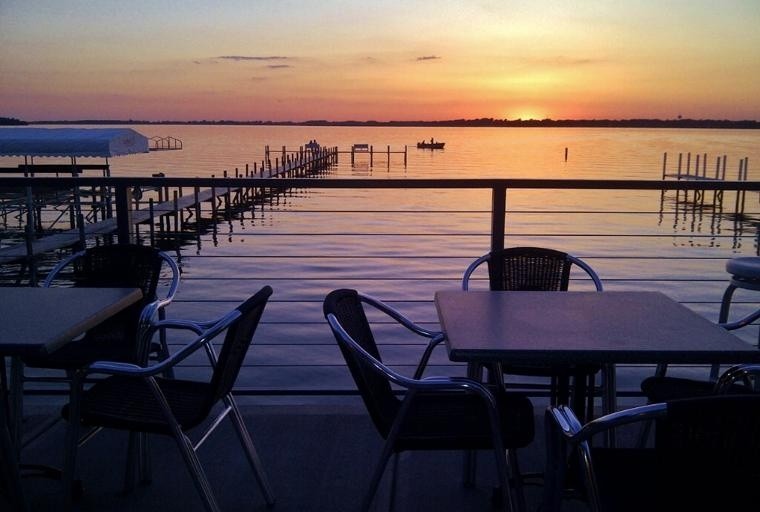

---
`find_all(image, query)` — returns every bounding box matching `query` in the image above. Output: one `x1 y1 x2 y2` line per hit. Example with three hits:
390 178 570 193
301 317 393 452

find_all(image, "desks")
434 292 753 484
0 286 140 512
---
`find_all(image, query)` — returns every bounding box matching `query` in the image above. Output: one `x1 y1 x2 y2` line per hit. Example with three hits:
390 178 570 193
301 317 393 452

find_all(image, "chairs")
322 288 537 509
39 245 179 495
545 392 758 510
632 308 760 453
61 285 276 511
461 247 618 495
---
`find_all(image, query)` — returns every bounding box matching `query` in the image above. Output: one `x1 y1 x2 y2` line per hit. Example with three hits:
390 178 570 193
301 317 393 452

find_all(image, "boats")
417 141 445 149
